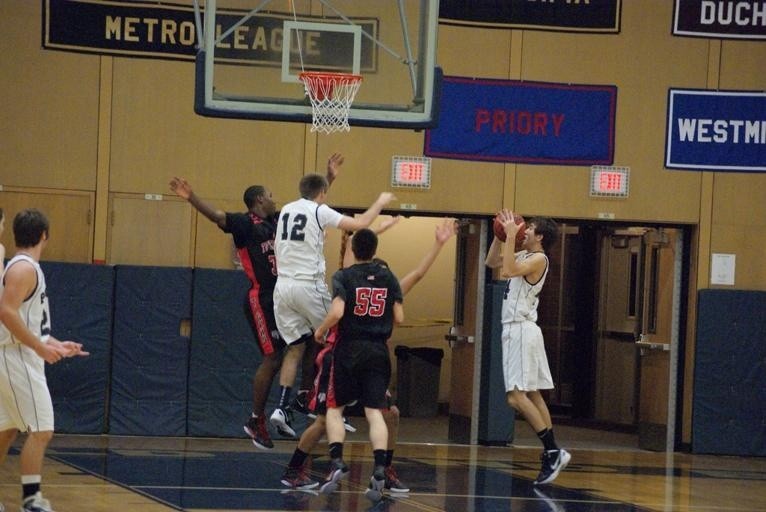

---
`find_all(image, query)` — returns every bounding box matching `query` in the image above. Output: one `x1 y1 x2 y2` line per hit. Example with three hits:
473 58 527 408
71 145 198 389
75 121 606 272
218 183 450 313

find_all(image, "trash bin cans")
395 344 445 419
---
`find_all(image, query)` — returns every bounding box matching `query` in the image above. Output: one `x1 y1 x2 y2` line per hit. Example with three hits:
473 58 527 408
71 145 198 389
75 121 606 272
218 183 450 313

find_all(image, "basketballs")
493 213 526 243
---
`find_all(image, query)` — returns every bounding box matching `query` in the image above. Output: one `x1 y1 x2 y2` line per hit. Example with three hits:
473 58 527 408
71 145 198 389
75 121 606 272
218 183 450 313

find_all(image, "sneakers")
537 448 571 484
270 408 296 438
364 465 386 502
243 412 275 451
19 491 55 512
318 459 350 494
342 416 357 432
280 470 319 490
384 468 410 492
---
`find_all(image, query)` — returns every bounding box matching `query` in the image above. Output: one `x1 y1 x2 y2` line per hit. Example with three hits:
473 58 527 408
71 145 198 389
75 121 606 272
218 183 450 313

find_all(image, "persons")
483 207 573 486
167 152 346 451
314 229 412 494
280 213 458 494
0 209 91 511
272 173 399 440
